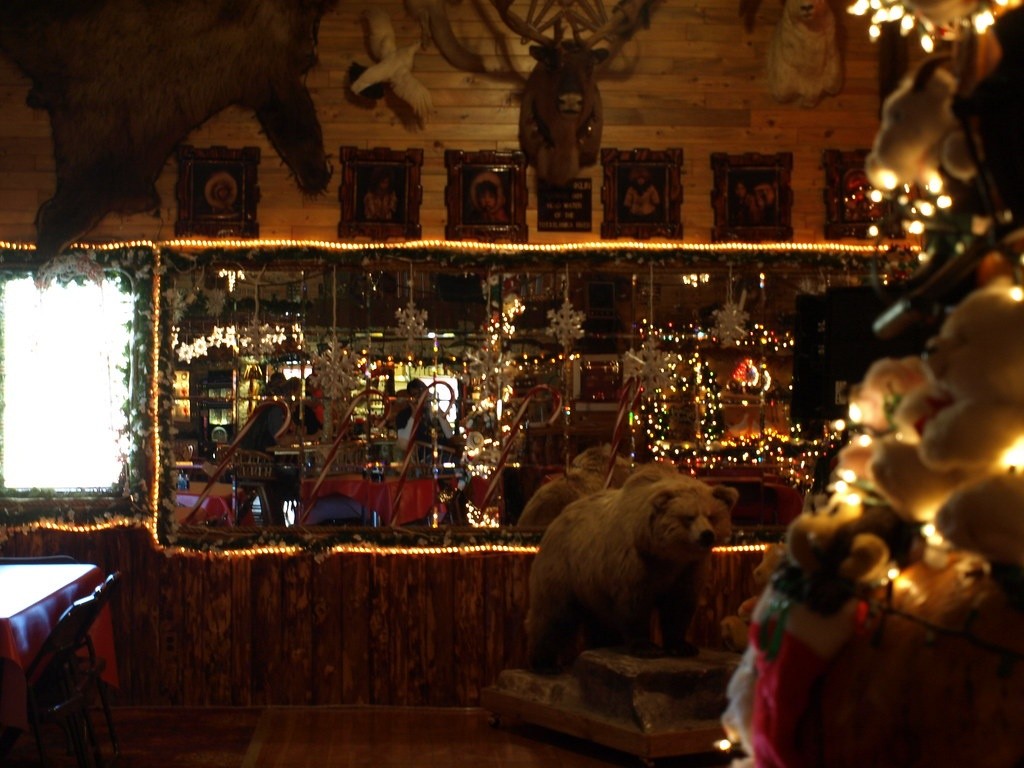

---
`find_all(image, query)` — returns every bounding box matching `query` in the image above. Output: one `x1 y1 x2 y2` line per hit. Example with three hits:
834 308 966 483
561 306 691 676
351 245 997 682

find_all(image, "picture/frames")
443 148 529 242
598 146 686 241
171 145 264 239
337 144 427 243
573 353 621 412
821 146 898 239
710 149 796 244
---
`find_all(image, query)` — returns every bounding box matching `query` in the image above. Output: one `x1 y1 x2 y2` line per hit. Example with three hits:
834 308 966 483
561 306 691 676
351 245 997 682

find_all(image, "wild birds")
351 4 437 124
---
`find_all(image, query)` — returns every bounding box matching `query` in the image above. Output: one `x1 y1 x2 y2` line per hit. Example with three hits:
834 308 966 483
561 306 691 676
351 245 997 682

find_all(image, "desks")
2 562 122 755
170 480 257 527
300 459 505 524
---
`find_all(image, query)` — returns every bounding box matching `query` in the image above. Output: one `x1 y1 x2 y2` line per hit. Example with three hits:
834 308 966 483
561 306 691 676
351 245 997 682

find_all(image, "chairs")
1 556 92 761
158 437 490 546
27 570 122 766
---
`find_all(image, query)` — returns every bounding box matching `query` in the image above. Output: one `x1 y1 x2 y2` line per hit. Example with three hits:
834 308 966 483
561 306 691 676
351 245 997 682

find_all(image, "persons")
241 369 474 526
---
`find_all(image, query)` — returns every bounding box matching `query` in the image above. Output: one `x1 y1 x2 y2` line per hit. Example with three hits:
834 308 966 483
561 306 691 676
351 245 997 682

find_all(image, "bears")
515 444 642 544
522 466 740 680
